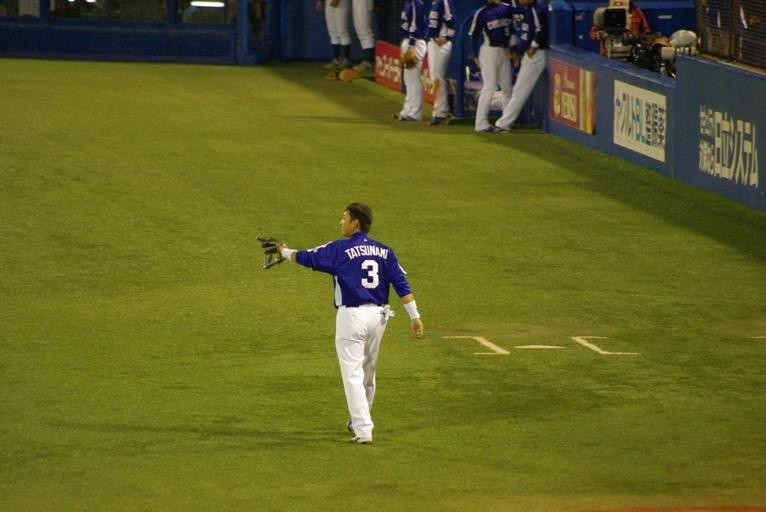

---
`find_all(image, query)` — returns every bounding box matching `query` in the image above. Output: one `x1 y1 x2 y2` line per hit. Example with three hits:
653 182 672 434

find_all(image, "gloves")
382 304 395 321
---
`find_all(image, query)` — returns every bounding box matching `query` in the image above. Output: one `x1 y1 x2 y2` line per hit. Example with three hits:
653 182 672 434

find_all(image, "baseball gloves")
400 46 419 68
255 234 285 270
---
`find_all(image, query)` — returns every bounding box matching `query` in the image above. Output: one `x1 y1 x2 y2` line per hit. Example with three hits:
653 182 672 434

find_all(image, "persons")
315 0 352 81
350 0 375 77
483 0 549 132
468 0 518 134
666 28 717 83
588 0 650 64
254 202 425 445
426 0 459 127
392 0 429 122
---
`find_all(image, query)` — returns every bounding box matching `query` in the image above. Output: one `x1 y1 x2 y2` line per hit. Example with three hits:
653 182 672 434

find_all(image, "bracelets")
280 248 297 261
402 299 420 320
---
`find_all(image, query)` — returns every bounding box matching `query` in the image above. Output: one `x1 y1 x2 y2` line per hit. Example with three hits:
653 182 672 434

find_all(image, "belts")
345 302 382 308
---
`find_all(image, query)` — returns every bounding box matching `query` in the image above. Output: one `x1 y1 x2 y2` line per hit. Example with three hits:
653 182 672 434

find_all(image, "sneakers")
325 57 375 79
346 422 372 445
394 114 511 134
